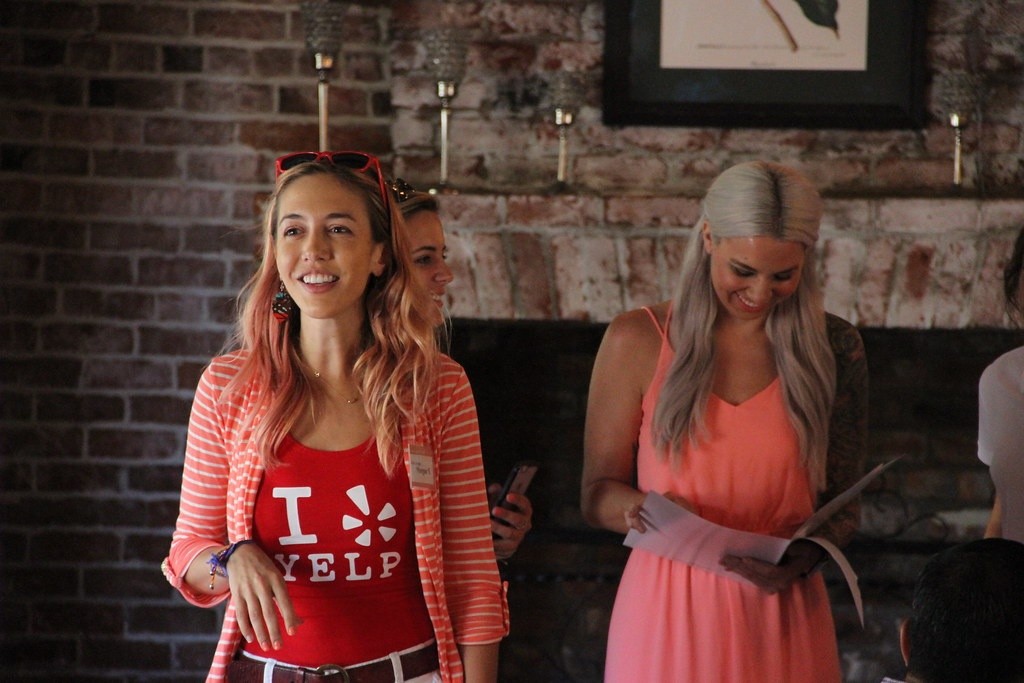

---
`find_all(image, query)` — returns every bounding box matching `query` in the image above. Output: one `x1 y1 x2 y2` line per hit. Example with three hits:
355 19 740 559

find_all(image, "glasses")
275 150 390 219
382 178 417 203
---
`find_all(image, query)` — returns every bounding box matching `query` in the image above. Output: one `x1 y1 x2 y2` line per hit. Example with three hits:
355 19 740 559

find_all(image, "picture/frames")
599 0 936 133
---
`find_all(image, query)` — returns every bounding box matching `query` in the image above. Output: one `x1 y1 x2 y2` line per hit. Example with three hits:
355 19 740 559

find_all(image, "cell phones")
489 461 540 542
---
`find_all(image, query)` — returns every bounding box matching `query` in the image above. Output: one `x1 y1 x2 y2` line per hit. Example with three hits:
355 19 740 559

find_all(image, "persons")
976 224 1024 540
876 538 1024 683
160 146 509 683
393 187 536 562
578 163 863 683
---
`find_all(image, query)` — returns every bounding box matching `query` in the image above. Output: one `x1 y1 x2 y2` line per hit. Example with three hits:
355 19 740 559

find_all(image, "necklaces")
302 359 365 404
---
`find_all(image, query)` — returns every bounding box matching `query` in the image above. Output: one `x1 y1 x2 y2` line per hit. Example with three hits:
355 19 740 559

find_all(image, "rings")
514 519 528 531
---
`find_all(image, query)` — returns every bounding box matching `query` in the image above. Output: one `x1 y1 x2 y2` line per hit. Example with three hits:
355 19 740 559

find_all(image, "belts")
227 639 438 683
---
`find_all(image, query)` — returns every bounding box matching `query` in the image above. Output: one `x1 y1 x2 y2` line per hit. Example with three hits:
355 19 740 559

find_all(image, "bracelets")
205 539 255 591
